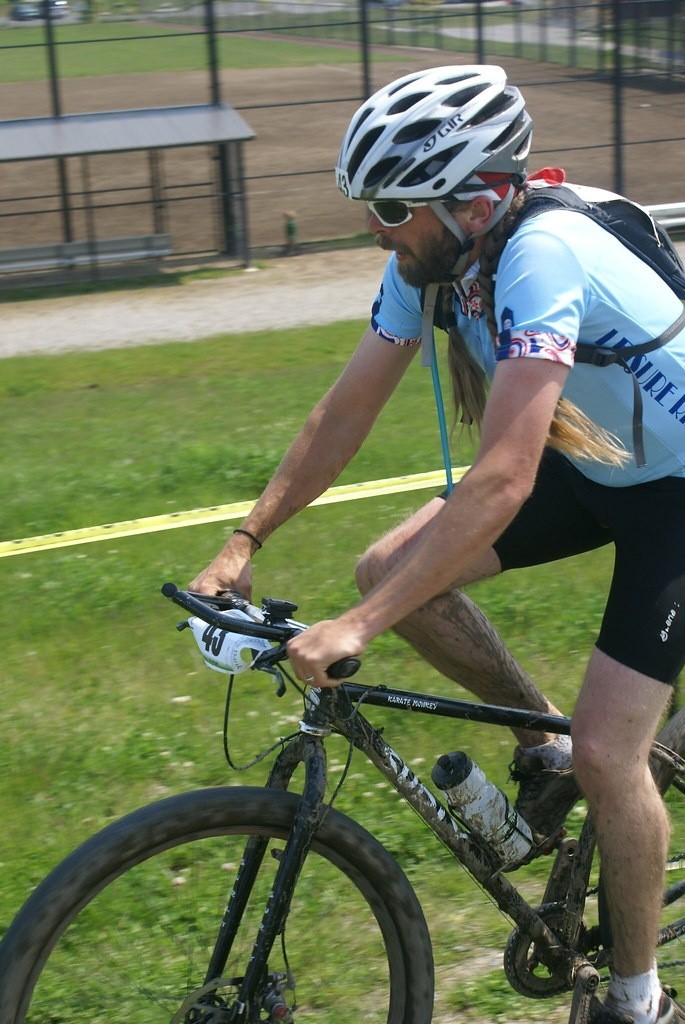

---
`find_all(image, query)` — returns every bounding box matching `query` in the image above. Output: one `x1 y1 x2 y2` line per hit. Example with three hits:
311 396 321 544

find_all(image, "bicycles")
0 582 685 1024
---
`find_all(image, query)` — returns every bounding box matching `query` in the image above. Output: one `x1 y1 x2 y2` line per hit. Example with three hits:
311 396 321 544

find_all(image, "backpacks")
420 175 685 367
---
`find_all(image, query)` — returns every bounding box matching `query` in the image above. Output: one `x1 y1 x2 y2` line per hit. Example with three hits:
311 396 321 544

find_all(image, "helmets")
335 64 534 200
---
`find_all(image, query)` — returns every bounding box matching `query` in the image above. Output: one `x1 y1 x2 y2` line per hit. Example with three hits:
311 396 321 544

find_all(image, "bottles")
431 751 533 864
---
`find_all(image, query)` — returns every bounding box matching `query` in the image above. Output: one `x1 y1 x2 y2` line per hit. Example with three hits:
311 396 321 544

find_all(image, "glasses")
366 200 450 228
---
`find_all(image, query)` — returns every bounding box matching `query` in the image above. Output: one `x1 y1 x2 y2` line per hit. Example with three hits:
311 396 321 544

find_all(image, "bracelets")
232 529 262 551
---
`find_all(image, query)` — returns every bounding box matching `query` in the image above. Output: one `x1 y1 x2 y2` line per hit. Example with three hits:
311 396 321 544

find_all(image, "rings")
305 676 313 682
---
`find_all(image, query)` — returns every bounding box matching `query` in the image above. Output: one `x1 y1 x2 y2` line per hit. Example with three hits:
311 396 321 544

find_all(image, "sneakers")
498 744 584 874
587 985 685 1024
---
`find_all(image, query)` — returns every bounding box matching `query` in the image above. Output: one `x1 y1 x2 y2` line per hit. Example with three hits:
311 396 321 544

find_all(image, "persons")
186 64 685 1023
